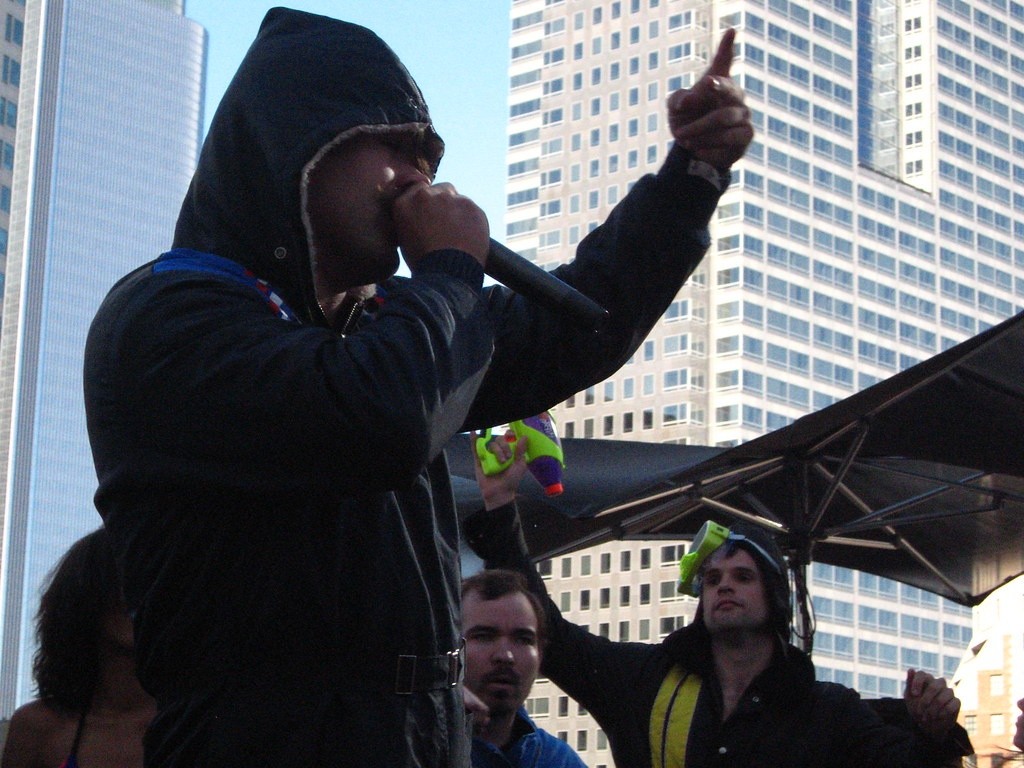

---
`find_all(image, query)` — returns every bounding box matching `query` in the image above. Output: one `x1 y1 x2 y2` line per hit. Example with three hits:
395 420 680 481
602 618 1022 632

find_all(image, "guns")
475 409 567 499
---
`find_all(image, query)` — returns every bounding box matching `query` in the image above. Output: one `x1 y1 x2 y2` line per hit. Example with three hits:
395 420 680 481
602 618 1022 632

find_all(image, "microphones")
484 238 610 338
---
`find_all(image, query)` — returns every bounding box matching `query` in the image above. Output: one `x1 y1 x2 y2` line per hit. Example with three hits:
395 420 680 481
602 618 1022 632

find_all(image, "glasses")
678 521 730 598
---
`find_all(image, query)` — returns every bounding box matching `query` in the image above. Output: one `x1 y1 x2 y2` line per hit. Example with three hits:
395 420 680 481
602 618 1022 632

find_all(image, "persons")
461 431 974 768
83 7 755 768
456 569 588 768
0 527 157 768
